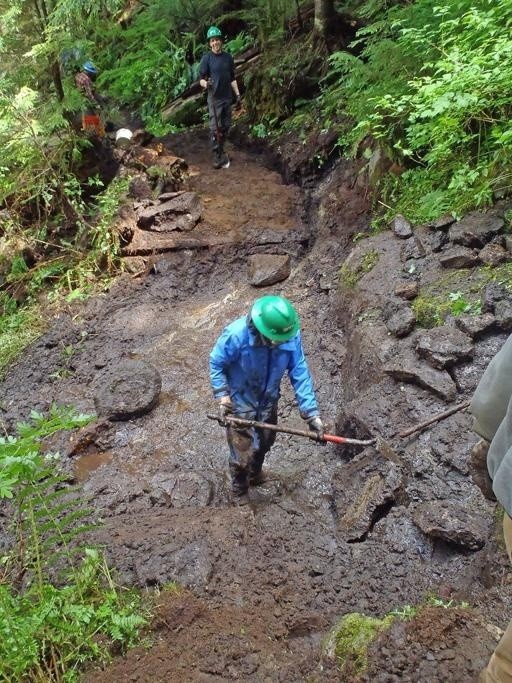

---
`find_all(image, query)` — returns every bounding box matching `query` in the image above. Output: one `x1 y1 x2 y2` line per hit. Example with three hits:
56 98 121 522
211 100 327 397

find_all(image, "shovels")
204 78 230 169
207 414 402 464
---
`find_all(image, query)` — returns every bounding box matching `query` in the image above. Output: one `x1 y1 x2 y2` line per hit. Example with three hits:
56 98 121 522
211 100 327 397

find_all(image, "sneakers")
213 160 220 169
228 153 233 161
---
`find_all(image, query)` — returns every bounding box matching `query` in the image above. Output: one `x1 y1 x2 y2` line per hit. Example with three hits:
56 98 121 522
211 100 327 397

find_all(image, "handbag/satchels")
82 115 100 129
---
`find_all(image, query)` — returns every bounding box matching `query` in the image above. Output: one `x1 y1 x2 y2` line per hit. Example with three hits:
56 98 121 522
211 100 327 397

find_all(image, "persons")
75 62 101 129
198 26 243 169
208 295 329 508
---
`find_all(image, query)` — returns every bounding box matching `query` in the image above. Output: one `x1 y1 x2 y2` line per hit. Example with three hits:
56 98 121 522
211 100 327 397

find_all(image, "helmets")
207 26 223 40
250 295 300 343
82 61 97 75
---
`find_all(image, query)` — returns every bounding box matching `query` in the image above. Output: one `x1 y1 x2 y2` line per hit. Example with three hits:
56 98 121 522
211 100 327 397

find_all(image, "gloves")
206 80 214 91
304 415 328 448
217 402 238 428
232 94 241 111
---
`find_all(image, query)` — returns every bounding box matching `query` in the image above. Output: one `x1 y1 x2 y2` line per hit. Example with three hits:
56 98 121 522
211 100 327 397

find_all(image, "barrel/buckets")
115 128 133 150
